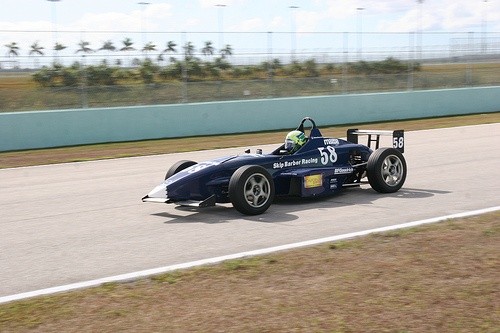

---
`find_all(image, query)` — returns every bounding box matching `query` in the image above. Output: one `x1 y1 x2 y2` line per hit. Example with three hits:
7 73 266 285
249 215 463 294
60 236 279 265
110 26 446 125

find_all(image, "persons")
284 130 306 155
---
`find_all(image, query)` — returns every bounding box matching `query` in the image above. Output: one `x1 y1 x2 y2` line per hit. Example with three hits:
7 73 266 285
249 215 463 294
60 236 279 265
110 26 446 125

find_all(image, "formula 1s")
141 117 408 216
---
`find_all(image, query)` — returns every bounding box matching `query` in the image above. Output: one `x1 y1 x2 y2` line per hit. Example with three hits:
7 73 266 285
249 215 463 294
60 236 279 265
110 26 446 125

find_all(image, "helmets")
284 130 307 151
